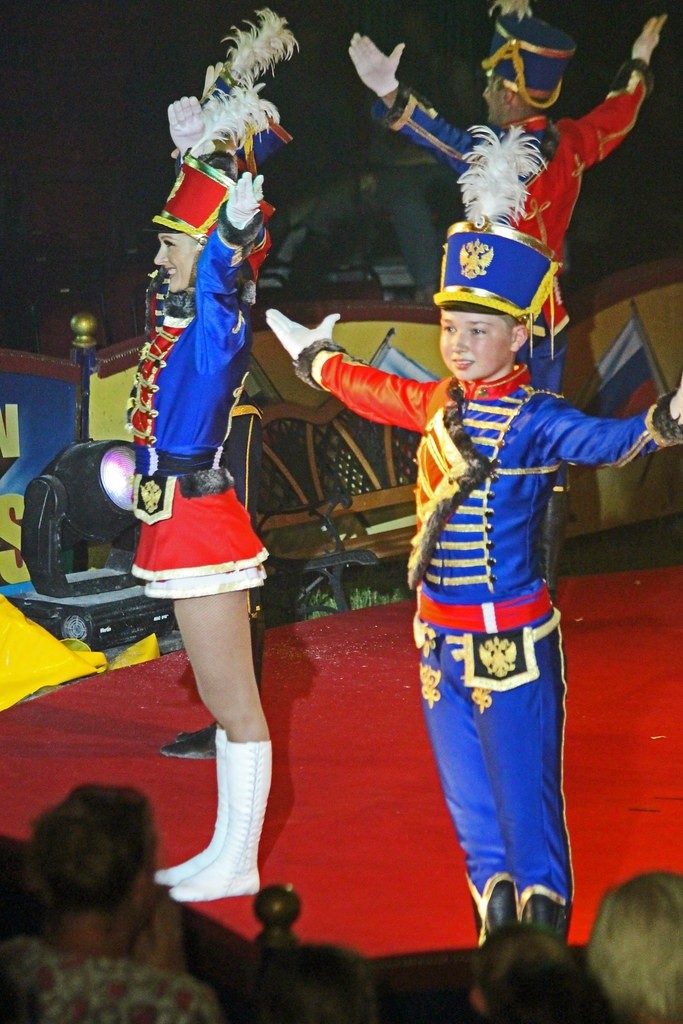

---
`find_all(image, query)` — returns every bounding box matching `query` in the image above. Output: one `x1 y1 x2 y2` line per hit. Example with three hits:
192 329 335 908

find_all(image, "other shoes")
159 722 216 760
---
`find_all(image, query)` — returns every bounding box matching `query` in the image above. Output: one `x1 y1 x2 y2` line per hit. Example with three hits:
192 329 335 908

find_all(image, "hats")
141 75 280 248
432 124 564 360
199 7 300 179
481 0 577 109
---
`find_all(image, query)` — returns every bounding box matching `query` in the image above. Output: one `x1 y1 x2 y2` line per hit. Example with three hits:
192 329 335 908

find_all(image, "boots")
464 868 573 948
154 728 273 902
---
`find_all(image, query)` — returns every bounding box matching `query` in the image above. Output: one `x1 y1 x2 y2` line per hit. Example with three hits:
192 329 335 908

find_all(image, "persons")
130 7 302 904
1 781 683 1022
264 123 683 951
348 0 668 608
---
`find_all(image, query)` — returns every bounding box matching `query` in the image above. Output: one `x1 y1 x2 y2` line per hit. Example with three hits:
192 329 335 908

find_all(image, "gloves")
167 96 206 163
226 172 266 231
630 14 668 66
202 62 267 97
669 377 683 431
348 32 406 97
264 308 341 391
